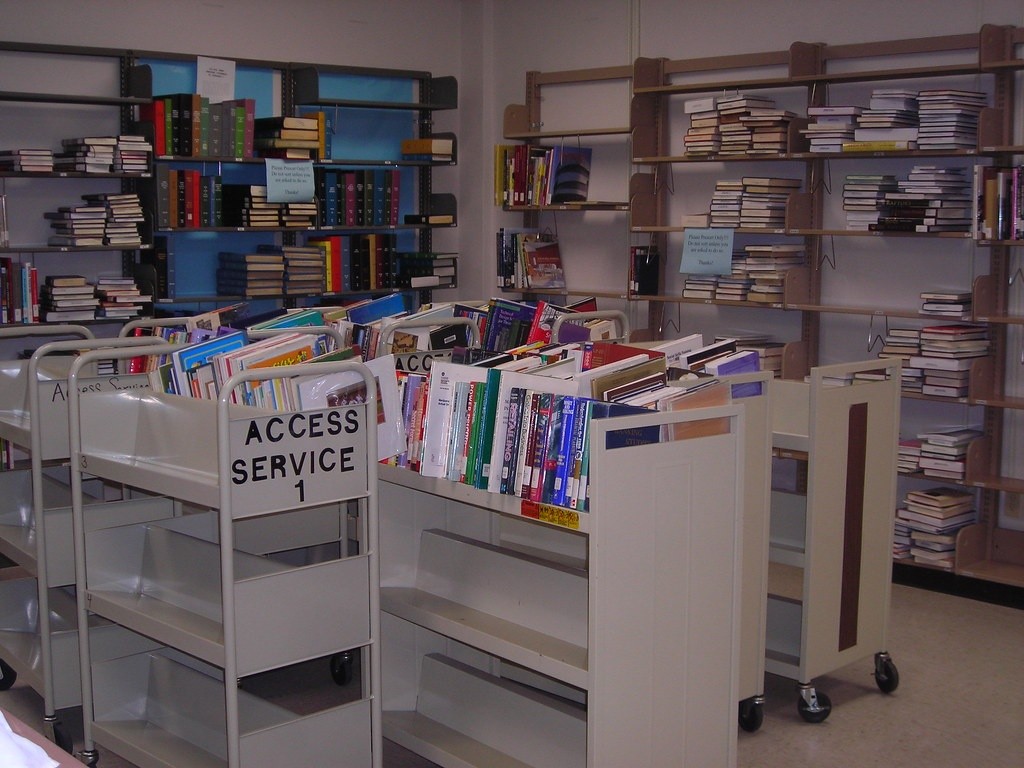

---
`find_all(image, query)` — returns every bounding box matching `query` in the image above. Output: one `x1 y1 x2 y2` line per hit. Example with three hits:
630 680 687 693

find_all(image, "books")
799 89 1024 569
0 91 798 511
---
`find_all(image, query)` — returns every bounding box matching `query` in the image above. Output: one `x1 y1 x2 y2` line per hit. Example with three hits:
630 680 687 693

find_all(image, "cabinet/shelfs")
0 25 1024 768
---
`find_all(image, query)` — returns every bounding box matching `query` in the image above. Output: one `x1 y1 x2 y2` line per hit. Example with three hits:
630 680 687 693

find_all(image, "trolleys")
1 317 899 768
